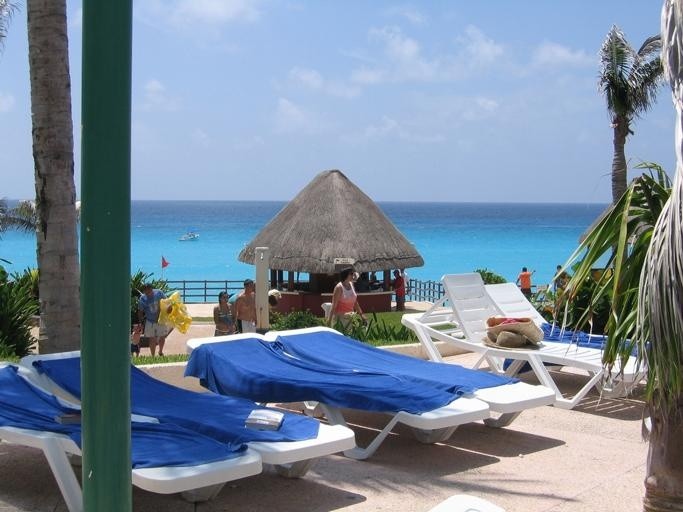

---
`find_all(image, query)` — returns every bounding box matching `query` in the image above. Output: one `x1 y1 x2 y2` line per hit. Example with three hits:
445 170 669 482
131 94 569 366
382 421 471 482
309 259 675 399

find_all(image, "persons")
138 283 172 359
392 270 410 311
131 323 144 358
233 278 256 333
515 267 536 302
214 291 237 336
359 270 378 293
554 265 572 292
327 269 367 334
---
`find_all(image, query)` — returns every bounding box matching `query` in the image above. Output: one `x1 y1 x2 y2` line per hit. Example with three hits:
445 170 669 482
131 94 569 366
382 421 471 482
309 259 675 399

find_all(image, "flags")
162 255 172 268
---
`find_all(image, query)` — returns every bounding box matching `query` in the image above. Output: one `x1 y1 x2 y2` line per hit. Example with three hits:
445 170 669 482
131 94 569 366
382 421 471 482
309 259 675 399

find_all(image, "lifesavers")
158 292 192 333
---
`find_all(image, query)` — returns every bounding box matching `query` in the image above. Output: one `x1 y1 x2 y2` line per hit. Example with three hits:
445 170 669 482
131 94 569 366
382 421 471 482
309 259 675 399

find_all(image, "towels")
275 332 518 395
31 356 321 447
503 323 647 375
184 339 463 413
0 366 249 471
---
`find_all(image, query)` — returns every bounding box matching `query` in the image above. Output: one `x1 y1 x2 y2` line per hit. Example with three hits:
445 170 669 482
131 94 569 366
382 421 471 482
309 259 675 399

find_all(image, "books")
245 408 285 431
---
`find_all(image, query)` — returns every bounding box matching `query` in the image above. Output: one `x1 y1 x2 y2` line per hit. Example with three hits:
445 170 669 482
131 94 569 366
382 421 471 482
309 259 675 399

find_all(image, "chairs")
183 331 490 460
401 273 648 410
264 323 557 428
485 282 651 397
20 351 357 479
1 362 264 512
